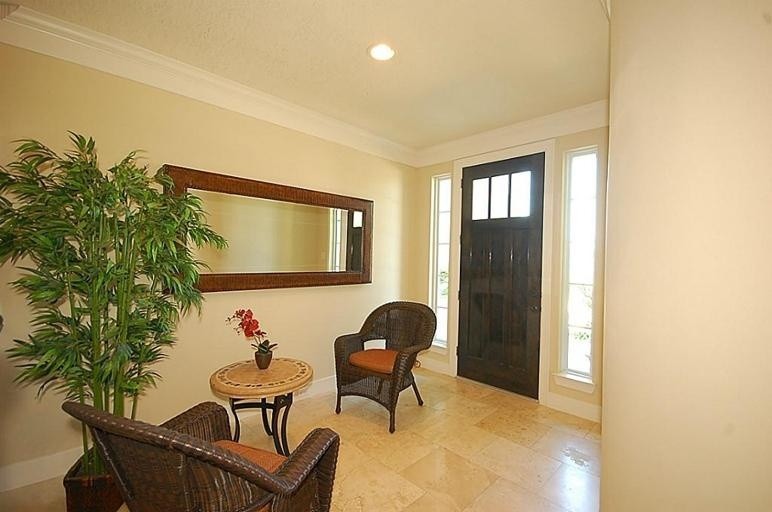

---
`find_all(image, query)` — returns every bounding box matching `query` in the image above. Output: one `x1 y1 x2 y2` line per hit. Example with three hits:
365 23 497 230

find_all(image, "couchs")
62 401 340 512
335 302 437 434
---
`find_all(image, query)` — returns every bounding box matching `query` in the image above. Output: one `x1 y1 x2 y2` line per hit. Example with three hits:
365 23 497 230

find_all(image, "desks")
210 359 314 457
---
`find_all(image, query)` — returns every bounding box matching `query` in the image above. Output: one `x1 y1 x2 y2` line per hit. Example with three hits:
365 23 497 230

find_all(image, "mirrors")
163 164 374 294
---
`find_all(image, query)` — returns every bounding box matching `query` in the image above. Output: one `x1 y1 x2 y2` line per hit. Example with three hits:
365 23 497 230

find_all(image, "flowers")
225 308 277 353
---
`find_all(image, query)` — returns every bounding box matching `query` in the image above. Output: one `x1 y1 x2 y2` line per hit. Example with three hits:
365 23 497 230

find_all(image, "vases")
256 355 272 368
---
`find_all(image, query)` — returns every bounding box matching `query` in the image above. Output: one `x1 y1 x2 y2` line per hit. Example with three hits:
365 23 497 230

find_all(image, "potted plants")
0 130 228 512
